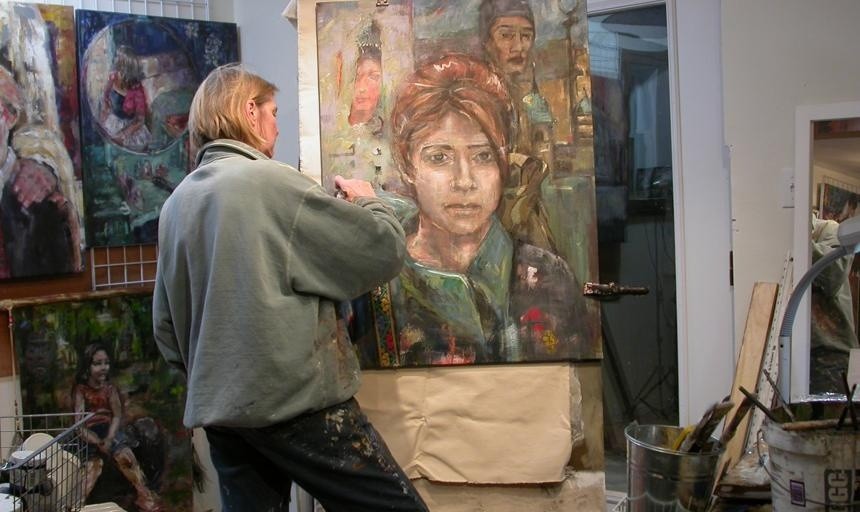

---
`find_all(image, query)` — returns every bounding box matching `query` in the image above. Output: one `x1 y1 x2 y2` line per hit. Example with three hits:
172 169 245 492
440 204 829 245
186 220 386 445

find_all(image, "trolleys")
0 414 96 512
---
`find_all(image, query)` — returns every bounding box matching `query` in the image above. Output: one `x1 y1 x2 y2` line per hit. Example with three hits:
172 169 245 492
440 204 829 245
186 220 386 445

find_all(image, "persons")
150 66 429 512
812 203 855 396
100 44 151 148
75 347 163 512
0 2 77 282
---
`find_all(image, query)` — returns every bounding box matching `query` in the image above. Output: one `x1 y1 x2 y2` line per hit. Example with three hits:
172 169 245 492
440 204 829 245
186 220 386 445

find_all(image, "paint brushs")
678 391 755 453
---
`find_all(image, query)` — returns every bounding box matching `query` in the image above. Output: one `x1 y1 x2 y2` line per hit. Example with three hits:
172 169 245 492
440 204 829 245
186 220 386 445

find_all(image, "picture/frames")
789 101 859 408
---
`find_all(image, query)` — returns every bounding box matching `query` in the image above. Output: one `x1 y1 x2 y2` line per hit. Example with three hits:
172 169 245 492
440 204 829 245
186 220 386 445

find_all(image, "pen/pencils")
335 188 346 200
738 369 858 433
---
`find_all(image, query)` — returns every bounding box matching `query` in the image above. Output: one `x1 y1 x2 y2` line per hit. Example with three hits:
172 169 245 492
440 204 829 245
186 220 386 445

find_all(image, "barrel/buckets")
756 399 859 512
623 419 725 511
